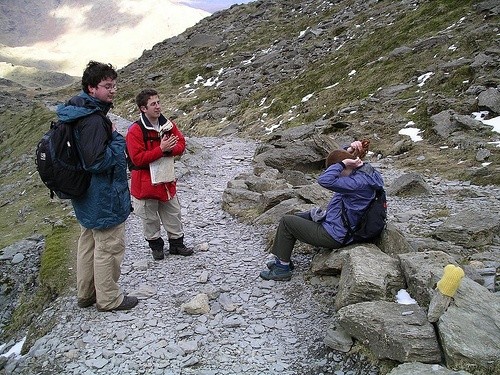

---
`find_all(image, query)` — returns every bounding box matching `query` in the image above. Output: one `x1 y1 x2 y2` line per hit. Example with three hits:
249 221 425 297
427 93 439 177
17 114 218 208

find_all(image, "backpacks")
35 112 110 197
341 189 387 242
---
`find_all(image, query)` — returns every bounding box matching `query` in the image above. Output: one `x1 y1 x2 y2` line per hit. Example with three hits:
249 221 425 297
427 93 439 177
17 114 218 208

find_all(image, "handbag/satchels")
149 156 175 185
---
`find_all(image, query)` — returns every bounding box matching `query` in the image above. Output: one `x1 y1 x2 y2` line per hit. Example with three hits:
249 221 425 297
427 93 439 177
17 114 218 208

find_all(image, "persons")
124 88 194 261
56 61 139 312
259 136 384 281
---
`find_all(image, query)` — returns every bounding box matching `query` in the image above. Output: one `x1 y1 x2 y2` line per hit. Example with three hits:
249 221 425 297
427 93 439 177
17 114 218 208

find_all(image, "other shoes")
110 296 138 311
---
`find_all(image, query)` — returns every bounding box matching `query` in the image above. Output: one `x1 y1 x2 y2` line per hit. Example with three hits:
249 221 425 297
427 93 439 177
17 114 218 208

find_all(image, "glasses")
98 84 117 91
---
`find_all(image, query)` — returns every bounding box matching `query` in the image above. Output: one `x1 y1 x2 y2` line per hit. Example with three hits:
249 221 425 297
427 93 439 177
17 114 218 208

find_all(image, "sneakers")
267 258 294 271
260 261 291 281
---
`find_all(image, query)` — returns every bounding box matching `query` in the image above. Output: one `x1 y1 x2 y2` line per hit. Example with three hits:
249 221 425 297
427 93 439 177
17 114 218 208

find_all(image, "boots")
145 237 164 260
169 234 192 256
